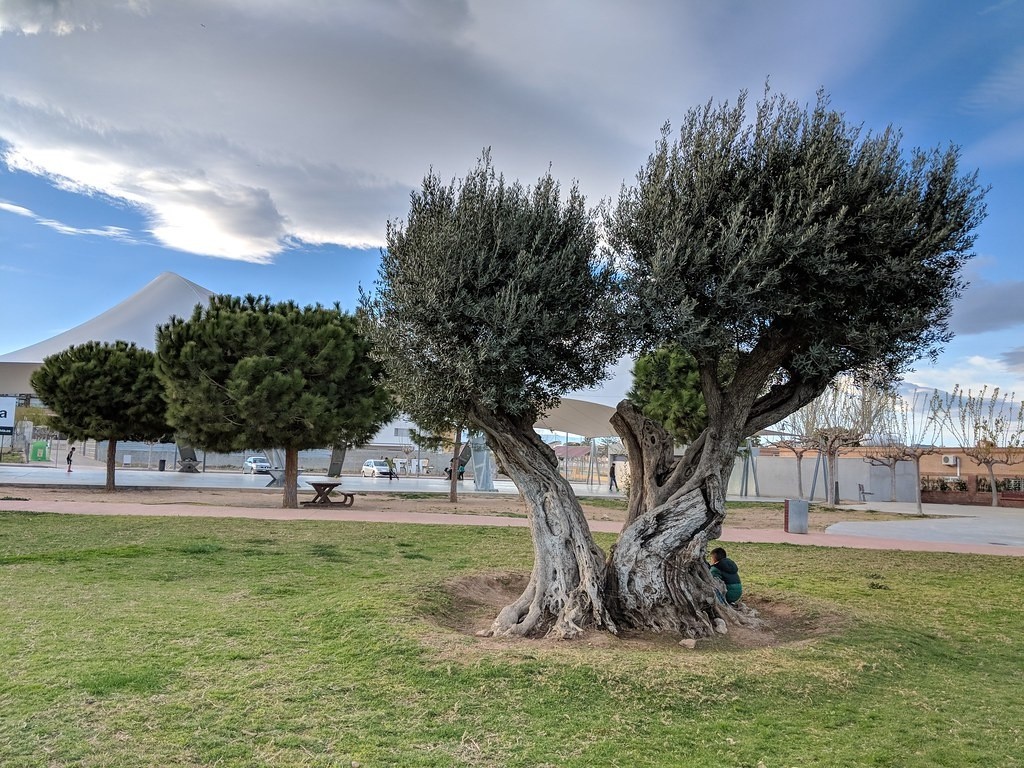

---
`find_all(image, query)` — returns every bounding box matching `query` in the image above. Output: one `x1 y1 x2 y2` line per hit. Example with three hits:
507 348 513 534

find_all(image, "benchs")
338 490 356 507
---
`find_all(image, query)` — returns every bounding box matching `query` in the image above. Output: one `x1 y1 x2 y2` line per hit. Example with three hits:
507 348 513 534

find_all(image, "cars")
242 455 272 475
361 459 397 478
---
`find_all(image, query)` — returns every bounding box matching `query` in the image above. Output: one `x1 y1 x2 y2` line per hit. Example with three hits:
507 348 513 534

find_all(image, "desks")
306 479 342 507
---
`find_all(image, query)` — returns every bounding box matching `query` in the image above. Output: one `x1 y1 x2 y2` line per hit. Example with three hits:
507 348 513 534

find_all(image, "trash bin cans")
784 499 809 534
159 459 167 472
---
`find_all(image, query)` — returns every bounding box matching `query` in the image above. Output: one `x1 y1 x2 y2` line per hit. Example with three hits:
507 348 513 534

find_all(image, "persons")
609 463 620 491
444 464 464 479
710 548 742 607
66 447 75 472
383 457 399 480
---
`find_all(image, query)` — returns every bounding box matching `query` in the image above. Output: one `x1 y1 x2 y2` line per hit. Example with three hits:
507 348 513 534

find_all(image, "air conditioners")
942 455 957 466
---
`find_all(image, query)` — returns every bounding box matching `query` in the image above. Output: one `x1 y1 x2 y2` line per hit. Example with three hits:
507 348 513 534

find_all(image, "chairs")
122 455 132 467
